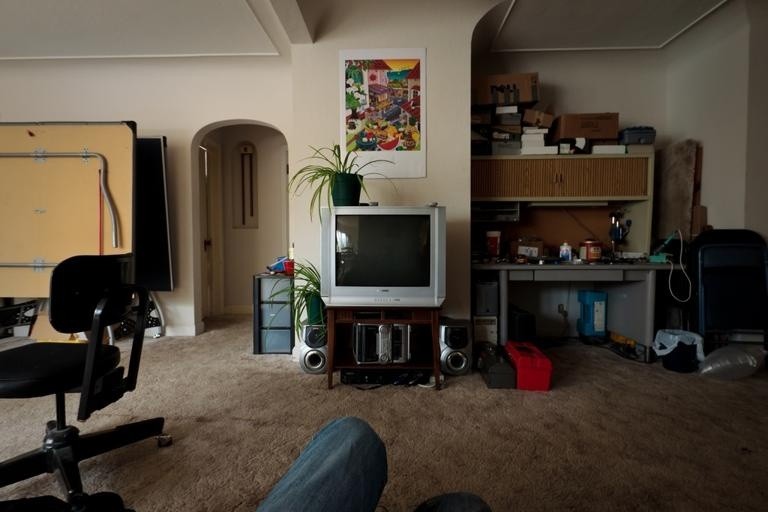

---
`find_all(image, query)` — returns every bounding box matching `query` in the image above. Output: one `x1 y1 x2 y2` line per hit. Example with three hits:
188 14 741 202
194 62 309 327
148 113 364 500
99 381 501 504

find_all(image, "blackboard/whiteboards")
136 136 175 292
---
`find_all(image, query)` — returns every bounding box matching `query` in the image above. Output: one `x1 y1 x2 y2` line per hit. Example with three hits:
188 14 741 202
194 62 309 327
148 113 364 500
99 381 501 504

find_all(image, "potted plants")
263 257 327 348
287 140 398 226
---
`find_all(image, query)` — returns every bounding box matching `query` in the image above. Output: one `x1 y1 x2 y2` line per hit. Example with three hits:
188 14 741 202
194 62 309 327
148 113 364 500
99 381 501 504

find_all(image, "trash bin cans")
657 329 704 374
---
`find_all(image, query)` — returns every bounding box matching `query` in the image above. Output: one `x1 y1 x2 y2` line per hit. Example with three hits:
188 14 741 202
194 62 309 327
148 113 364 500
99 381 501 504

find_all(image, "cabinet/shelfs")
473 154 652 200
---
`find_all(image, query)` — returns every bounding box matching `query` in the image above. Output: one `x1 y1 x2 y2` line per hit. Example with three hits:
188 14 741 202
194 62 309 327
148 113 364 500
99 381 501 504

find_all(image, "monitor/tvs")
320 207 447 311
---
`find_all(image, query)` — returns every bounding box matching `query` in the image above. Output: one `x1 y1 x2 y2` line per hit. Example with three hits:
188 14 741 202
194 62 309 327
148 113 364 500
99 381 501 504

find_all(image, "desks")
473 262 686 366
0 253 177 512
324 306 447 391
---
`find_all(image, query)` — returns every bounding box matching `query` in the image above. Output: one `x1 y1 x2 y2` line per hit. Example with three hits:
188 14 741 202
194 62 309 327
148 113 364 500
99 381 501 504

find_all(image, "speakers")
438 325 472 376
298 324 328 374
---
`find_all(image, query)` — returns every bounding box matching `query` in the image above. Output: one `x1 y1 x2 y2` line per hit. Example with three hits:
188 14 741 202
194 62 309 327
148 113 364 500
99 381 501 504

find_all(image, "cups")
486 231 501 257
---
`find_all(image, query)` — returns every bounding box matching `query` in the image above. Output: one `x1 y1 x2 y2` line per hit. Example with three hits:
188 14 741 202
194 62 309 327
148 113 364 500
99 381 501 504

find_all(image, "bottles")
559 241 572 262
